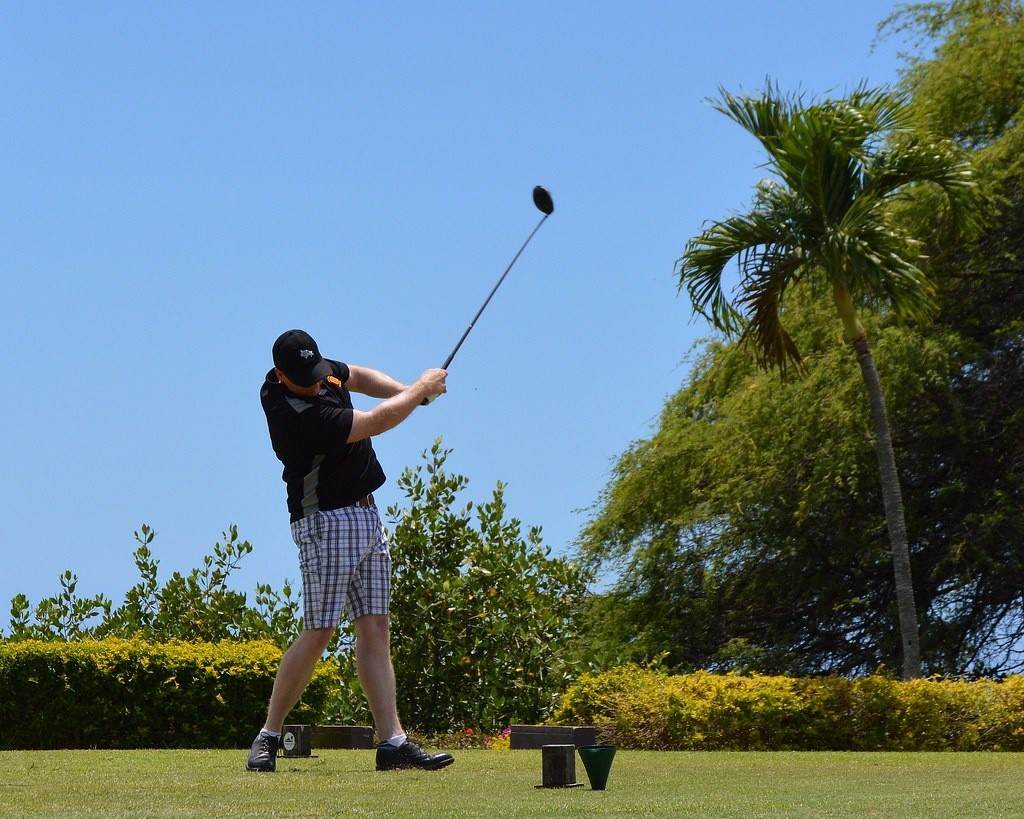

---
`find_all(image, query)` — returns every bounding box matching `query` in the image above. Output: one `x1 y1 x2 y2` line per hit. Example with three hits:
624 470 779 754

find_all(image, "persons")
247 329 457 773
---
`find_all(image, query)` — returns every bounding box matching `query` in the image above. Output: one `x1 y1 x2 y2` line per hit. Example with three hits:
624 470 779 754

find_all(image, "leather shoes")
246 731 282 772
375 735 455 770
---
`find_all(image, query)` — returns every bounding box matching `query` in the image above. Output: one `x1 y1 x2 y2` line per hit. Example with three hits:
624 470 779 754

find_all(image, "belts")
349 492 375 508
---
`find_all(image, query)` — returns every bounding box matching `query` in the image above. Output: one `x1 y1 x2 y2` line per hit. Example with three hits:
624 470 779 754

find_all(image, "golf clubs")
419 182 555 407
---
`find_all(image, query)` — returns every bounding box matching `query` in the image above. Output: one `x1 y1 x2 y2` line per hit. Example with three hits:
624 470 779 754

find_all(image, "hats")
271 329 333 387
